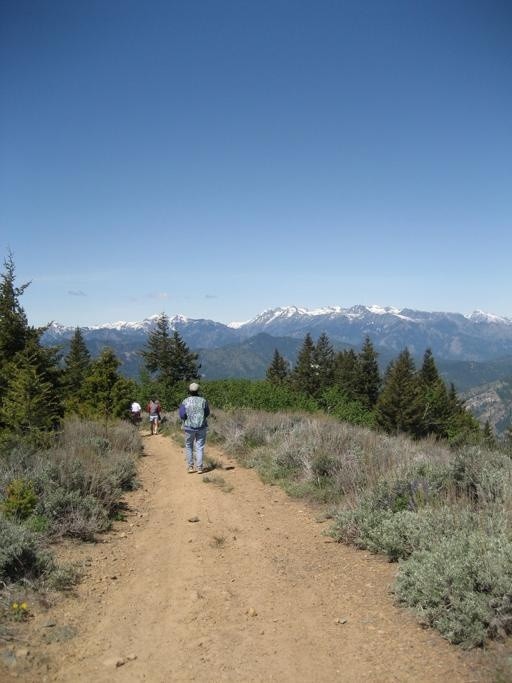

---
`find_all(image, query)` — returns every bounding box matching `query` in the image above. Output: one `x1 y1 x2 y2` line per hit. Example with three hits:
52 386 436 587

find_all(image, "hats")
189 383 199 391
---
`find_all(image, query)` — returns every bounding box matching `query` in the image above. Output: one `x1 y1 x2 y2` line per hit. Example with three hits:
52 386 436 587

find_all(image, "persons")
150 393 160 431
147 395 162 436
131 400 142 426
179 382 210 475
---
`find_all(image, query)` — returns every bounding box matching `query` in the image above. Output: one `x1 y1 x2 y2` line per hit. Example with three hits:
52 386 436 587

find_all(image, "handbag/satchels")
158 415 161 420
